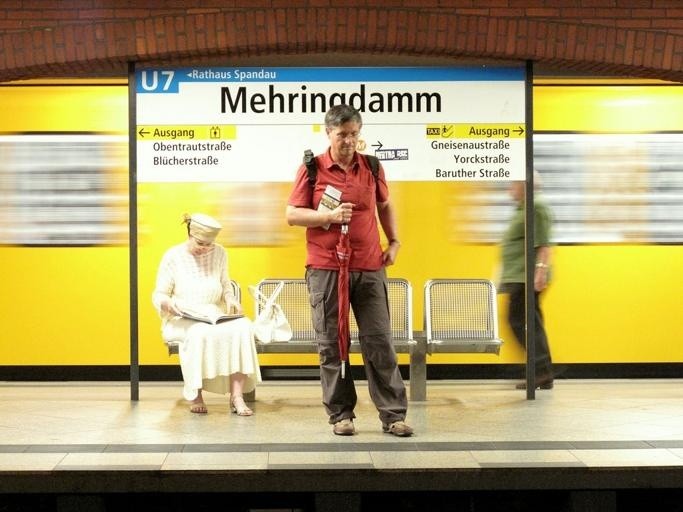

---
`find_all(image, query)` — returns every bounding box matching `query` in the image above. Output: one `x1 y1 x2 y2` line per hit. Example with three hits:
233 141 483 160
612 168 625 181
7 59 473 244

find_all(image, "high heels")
229 393 254 417
188 387 209 414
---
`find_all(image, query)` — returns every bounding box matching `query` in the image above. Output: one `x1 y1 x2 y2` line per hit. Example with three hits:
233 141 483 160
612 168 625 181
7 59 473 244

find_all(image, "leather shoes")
515 370 554 391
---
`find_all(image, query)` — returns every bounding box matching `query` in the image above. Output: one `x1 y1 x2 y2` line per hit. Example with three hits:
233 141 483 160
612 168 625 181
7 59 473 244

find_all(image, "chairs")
348 279 414 348
168 279 244 356
424 279 504 355
254 279 330 354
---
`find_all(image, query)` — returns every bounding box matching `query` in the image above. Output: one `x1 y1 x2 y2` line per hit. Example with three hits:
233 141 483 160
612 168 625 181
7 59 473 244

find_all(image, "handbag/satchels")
247 281 293 345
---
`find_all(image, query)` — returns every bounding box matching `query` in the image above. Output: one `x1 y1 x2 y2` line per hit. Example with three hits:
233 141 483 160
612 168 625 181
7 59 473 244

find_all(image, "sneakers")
331 414 357 436
381 420 415 436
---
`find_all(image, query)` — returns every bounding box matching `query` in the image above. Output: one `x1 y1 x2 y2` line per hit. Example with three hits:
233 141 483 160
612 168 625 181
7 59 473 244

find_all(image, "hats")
188 213 223 244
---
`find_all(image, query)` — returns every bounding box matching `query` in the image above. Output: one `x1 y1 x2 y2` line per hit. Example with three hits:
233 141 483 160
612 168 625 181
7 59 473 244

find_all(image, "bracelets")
534 262 548 269
388 239 401 246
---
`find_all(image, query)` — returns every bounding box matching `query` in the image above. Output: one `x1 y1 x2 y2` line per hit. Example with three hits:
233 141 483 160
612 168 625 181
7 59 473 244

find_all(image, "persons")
283 104 415 437
498 166 555 394
151 214 264 417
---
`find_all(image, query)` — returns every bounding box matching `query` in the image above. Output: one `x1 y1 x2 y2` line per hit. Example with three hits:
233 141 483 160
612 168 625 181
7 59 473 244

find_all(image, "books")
173 297 244 325
